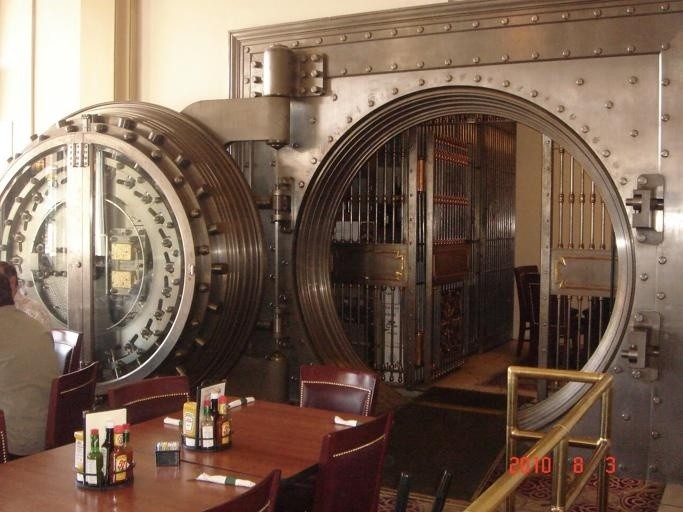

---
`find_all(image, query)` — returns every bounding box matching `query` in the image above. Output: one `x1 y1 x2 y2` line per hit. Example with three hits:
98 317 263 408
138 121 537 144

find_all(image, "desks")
1 442 268 512
129 395 380 482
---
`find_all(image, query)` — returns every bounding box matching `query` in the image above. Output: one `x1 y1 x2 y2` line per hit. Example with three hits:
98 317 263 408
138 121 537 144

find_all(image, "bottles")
84 422 135 486
196 392 233 448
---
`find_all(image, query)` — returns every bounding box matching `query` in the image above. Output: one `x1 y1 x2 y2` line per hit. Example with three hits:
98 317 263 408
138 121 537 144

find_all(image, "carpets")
377 386 535 502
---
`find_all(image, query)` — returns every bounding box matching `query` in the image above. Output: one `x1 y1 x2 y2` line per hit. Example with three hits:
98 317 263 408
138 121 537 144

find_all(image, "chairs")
45 361 100 451
51 328 83 376
205 468 283 512
514 265 581 370
108 376 191 424
0 410 11 464
315 412 393 512
299 364 379 417
